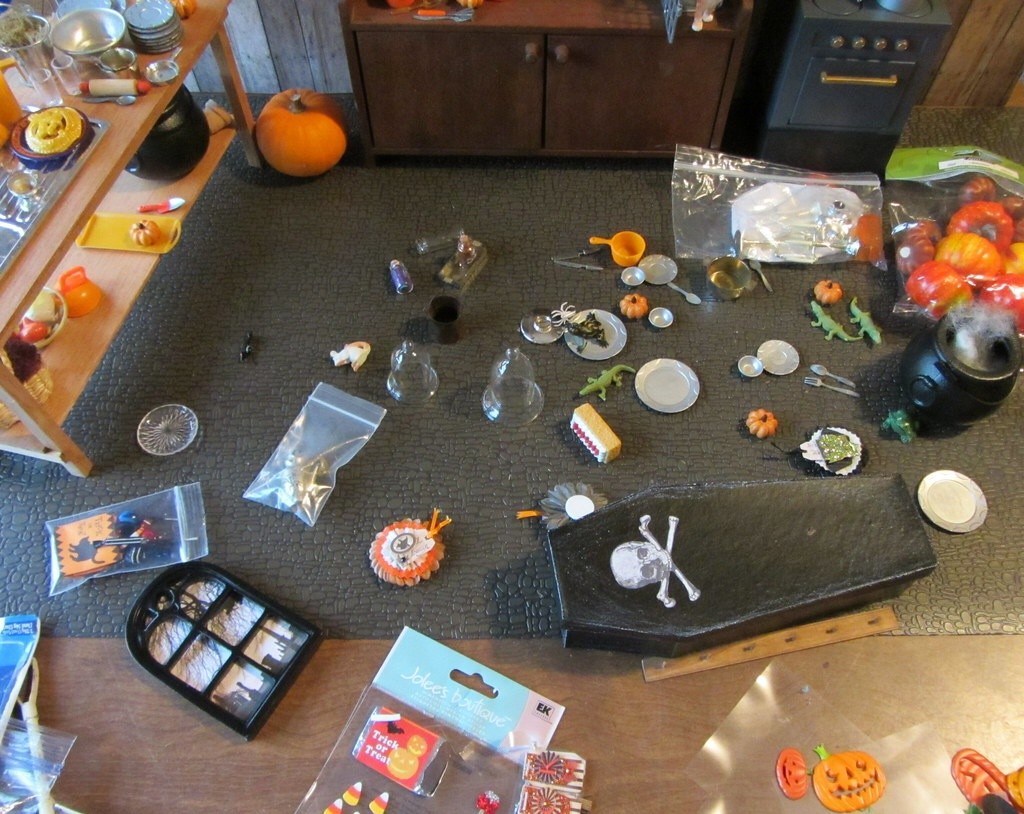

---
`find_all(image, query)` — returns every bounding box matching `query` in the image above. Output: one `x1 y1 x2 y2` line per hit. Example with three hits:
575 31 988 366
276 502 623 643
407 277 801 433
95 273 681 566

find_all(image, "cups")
50 56 84 95
428 295 464 345
28 69 64 107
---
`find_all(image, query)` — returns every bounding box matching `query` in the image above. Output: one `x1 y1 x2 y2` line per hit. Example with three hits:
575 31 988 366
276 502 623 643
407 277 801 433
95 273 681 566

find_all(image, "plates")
19 287 67 348
918 470 989 533
639 254 679 285
565 309 627 360
124 1 185 55
648 307 673 327
738 356 765 378
757 340 800 375
137 404 198 456
5 105 90 162
621 267 645 287
635 358 700 414
56 0 128 17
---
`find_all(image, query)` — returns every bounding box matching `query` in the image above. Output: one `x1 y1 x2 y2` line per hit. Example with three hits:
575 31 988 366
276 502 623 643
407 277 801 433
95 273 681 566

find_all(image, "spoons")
809 364 857 388
667 282 702 304
749 260 775 294
83 95 135 106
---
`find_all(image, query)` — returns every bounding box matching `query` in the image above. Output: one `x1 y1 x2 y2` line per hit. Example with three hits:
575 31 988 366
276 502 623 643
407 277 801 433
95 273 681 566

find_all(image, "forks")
805 376 860 398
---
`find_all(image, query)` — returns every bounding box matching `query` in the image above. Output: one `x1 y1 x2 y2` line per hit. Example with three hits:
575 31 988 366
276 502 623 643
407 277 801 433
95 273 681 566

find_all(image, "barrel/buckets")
48 7 127 72
1 14 57 84
95 47 141 80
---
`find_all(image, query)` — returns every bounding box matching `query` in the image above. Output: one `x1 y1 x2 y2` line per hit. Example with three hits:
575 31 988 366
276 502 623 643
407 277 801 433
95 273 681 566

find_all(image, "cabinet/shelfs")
333 0 748 171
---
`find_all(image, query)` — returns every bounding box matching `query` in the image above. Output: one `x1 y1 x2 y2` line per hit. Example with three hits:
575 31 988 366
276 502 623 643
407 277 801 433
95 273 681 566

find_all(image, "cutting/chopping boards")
75 211 182 255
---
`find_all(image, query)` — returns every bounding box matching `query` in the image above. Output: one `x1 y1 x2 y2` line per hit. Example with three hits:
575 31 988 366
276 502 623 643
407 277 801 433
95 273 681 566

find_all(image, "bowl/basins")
589 230 645 266
704 256 751 301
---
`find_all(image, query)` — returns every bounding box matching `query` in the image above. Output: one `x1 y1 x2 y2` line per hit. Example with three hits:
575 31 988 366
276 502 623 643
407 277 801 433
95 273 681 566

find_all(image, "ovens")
729 0 957 185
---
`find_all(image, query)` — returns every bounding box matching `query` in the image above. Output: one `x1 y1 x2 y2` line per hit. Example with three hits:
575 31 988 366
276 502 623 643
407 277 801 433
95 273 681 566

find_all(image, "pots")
141 46 184 85
896 309 1023 435
122 84 210 182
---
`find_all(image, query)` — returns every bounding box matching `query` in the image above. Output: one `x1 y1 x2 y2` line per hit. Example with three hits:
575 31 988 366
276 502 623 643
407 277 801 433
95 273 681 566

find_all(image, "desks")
0 1 263 484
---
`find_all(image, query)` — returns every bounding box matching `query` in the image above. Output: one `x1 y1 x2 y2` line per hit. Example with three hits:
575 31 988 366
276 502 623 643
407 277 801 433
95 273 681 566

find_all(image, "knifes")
137 198 187 214
554 261 604 272
550 246 601 261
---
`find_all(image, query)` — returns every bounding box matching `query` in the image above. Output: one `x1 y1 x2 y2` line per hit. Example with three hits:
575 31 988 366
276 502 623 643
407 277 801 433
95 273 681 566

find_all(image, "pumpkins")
255 87 353 183
890 202 1024 340
